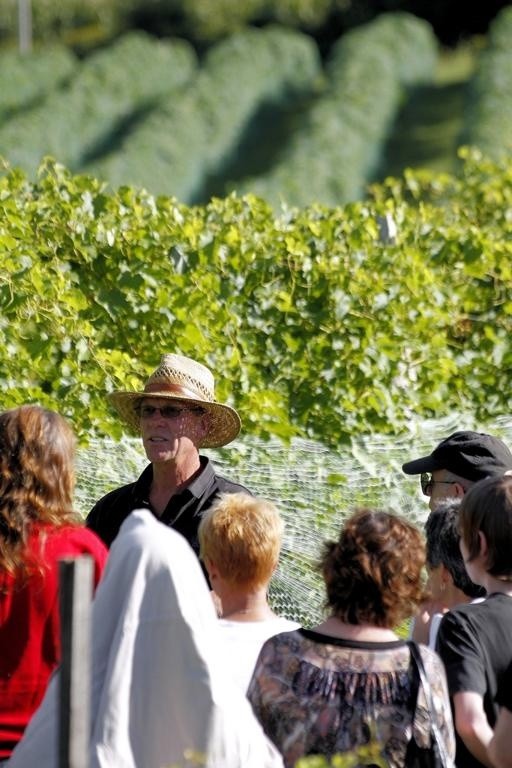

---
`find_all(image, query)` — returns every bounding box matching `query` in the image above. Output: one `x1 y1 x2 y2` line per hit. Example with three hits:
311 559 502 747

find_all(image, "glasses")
133 405 198 417
421 472 455 495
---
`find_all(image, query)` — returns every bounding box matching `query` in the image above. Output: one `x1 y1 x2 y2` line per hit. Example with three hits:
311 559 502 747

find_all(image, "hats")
108 353 242 447
403 431 512 482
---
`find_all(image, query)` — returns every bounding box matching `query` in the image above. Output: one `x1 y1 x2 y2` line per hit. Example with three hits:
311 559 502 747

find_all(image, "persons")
4 508 283 768
402 431 512 642
198 492 302 696
412 498 486 651
488 658 512 768
0 406 109 768
436 475 512 768
246 509 458 768
84 353 253 617
